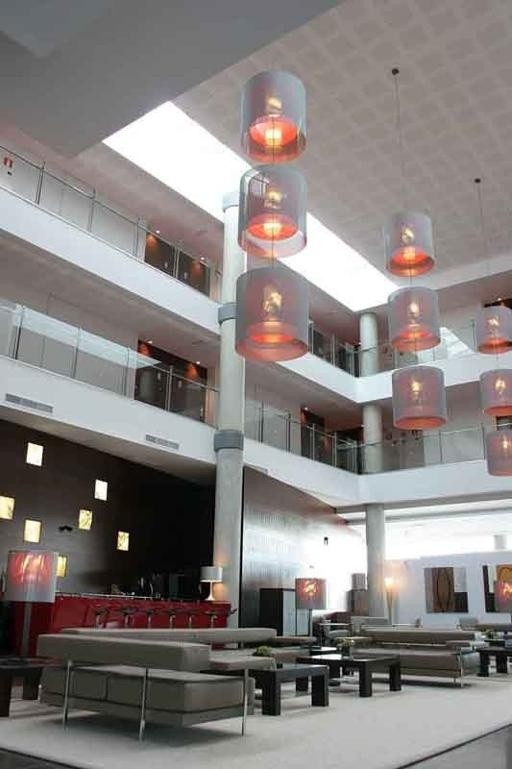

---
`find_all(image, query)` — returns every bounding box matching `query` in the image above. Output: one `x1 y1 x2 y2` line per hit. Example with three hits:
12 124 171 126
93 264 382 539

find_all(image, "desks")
201 665 330 715
295 652 403 697
459 619 512 677
0 657 45 717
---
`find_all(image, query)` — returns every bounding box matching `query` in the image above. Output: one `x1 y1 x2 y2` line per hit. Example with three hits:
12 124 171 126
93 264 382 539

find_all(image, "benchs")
40 634 276 741
63 626 316 664
332 628 489 688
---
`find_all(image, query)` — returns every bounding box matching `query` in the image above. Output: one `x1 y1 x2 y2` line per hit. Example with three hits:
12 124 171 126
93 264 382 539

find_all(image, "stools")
81 603 221 629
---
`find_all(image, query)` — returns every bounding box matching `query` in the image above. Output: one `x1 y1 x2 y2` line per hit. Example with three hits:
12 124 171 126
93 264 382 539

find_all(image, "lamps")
200 566 224 600
380 70 447 431
234 45 308 363
2 549 61 661
468 172 512 477
295 577 326 635
